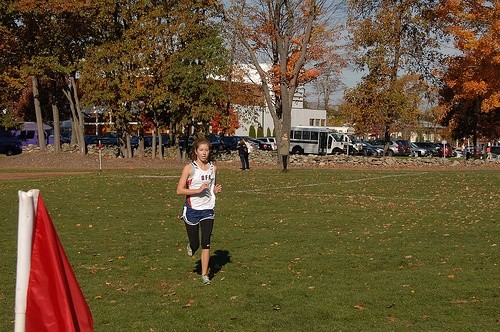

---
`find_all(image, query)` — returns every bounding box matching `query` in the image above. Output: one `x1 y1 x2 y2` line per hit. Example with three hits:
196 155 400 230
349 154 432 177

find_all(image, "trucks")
289 125 353 156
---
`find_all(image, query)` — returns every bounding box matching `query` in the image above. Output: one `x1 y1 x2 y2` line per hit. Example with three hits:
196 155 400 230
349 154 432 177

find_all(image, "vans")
10 122 54 147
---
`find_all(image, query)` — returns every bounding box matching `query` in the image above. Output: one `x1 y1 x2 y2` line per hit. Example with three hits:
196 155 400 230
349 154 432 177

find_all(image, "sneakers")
200 275 211 285
187 244 193 257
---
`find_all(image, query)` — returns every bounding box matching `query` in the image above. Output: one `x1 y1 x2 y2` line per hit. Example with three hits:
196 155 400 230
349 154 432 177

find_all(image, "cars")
172 135 277 155
48 133 175 149
351 140 453 158
0 136 22 157
452 145 500 161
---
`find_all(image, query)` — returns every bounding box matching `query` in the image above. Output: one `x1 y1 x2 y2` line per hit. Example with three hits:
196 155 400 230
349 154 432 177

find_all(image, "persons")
279 133 289 171
236 137 251 170
176 138 223 287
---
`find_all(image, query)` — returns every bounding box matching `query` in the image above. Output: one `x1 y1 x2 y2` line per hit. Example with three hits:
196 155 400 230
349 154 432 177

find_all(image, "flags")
25 186 94 332
